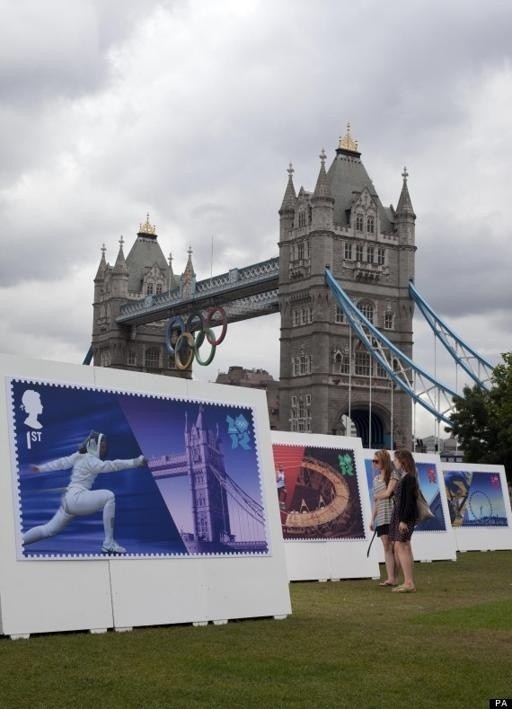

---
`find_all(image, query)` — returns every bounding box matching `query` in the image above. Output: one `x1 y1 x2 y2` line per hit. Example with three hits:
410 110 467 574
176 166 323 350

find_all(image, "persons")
370 450 399 586
276 464 288 505
388 450 419 593
23 430 145 554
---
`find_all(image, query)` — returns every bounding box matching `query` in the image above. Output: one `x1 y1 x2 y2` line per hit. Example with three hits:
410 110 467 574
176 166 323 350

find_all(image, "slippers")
378 582 397 586
393 585 417 593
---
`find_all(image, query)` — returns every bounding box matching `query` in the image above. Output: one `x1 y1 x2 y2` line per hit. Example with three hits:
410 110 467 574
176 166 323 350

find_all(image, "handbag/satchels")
414 489 435 527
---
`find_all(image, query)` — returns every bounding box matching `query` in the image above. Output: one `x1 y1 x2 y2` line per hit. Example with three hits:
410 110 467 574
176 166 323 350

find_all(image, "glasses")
373 460 379 464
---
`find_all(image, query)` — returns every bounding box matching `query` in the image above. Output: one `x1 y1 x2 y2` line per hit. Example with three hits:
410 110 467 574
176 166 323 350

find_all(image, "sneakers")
102 541 127 553
